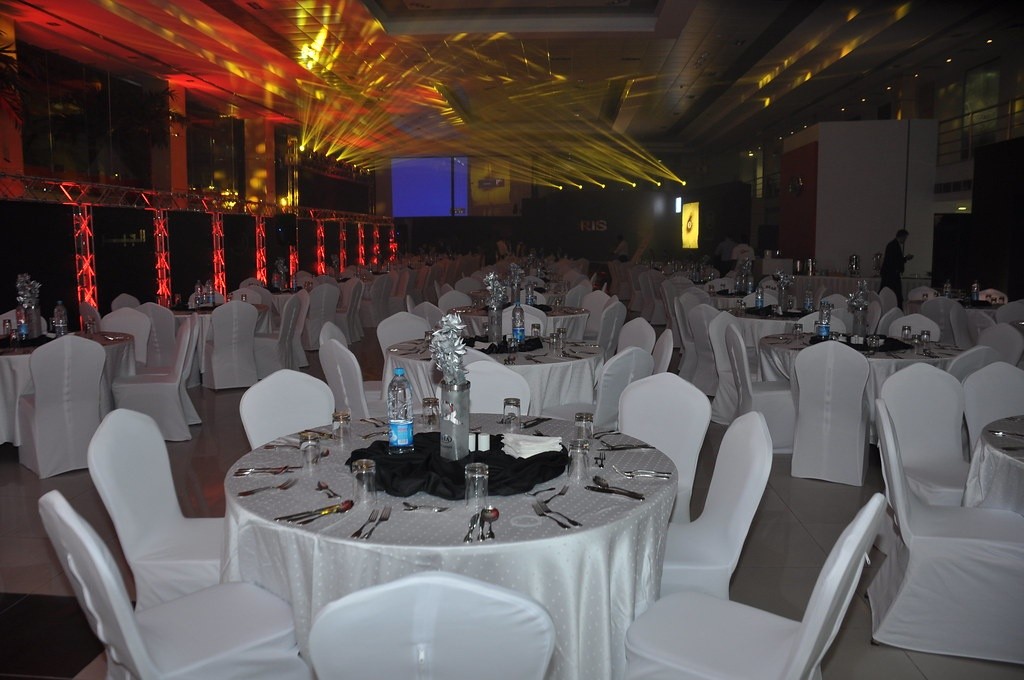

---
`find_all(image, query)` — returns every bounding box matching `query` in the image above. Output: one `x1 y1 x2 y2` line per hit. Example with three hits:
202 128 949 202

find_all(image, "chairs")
1 247 1024 679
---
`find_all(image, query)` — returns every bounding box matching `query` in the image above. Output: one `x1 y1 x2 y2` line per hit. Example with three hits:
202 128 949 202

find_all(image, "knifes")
351 429 672 542
464 513 479 544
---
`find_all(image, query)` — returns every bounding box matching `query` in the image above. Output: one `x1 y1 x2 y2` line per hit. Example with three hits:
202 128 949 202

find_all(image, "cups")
532 268 567 357
299 397 595 509
226 292 247 302
901 325 931 355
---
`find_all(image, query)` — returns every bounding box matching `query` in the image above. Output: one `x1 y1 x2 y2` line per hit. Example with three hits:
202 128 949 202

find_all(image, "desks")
0 333 135 446
380 337 605 415
717 308 806 348
167 303 270 373
758 333 967 445
710 293 750 311
446 307 589 342
223 409 678 679
907 299 1004 346
959 415 1024 514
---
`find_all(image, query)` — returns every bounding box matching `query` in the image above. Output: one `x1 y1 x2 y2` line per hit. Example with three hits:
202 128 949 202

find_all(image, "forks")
389 338 433 362
764 333 810 351
234 417 387 525
504 341 599 366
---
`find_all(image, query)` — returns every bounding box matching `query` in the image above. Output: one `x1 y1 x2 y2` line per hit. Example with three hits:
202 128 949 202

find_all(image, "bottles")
512 302 525 347
195 279 215 306
804 286 813 314
943 280 978 301
537 263 542 278
273 269 298 292
818 305 829 341
525 281 533 305
387 368 415 455
16 300 67 340
755 284 764 309
734 274 752 296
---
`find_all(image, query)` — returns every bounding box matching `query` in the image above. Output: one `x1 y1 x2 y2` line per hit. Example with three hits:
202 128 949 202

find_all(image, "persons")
613 234 631 263
716 231 756 276
878 229 915 312
